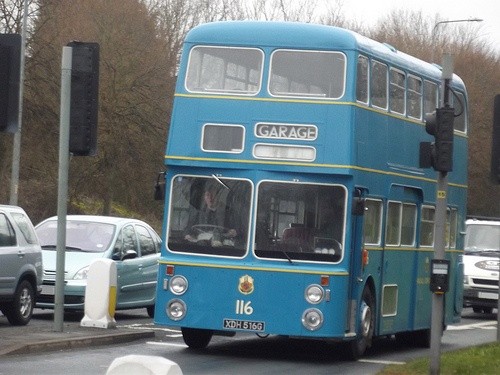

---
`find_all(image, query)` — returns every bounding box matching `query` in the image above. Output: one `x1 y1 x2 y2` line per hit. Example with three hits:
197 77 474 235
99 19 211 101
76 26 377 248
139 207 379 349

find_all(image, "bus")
151 22 469 360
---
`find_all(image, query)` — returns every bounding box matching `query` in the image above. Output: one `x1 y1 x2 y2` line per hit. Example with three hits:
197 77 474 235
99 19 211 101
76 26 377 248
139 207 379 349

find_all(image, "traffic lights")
417 106 455 173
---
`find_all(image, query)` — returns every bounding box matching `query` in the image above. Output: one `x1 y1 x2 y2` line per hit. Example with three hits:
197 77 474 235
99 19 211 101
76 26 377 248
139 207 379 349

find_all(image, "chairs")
283 228 314 249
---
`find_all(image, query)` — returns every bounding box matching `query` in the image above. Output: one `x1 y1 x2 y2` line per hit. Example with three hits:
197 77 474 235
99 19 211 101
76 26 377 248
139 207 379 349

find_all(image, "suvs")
0 205 44 323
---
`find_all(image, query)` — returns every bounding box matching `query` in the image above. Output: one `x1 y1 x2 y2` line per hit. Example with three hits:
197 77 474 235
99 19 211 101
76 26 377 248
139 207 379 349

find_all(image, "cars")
33 215 166 315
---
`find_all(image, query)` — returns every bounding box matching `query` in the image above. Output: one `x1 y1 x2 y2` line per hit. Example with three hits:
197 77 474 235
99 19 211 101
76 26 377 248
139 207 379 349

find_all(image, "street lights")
431 18 484 62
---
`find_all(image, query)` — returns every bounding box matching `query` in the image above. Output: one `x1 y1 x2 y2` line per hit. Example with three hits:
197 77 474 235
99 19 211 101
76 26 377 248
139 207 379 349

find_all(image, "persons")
177 182 245 245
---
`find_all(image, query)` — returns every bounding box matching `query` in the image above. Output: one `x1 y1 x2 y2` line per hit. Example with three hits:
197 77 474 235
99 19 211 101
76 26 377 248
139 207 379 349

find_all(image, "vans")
461 218 500 315
